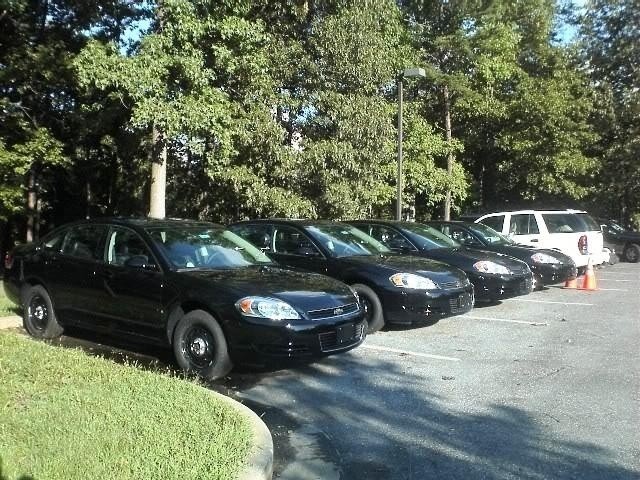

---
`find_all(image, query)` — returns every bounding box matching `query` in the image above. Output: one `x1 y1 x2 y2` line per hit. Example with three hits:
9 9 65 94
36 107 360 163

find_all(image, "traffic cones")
576 257 602 291
562 278 578 289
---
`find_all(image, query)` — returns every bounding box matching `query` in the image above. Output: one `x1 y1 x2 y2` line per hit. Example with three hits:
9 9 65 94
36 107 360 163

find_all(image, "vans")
474 208 605 278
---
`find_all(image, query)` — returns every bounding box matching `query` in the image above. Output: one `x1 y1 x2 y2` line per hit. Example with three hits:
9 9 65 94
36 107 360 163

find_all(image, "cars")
223 218 476 335
585 217 638 264
418 219 578 291
340 219 534 307
3 216 369 382
